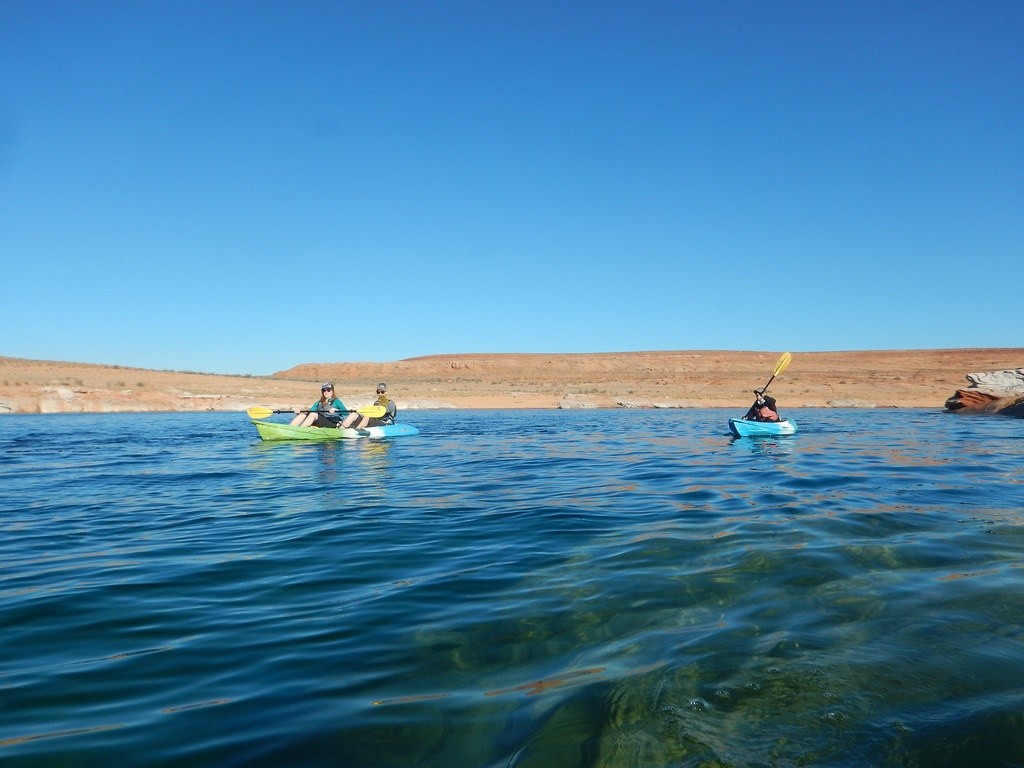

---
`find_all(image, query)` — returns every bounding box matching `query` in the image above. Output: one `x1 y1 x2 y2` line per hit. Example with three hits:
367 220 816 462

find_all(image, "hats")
378 383 386 390
321 383 334 391
754 388 766 394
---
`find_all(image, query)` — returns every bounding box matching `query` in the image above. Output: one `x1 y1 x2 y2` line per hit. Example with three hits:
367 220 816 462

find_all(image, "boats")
728 417 798 438
251 419 421 442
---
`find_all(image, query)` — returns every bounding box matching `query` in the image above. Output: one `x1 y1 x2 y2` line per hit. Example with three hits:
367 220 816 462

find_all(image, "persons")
339 383 396 429
289 382 350 428
742 388 781 422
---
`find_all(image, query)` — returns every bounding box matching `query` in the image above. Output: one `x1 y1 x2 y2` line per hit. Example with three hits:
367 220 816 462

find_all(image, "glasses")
323 388 331 392
377 391 386 394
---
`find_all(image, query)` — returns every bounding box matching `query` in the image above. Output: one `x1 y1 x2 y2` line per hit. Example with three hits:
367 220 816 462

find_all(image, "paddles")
247 406 386 418
377 395 388 406
745 352 792 418
